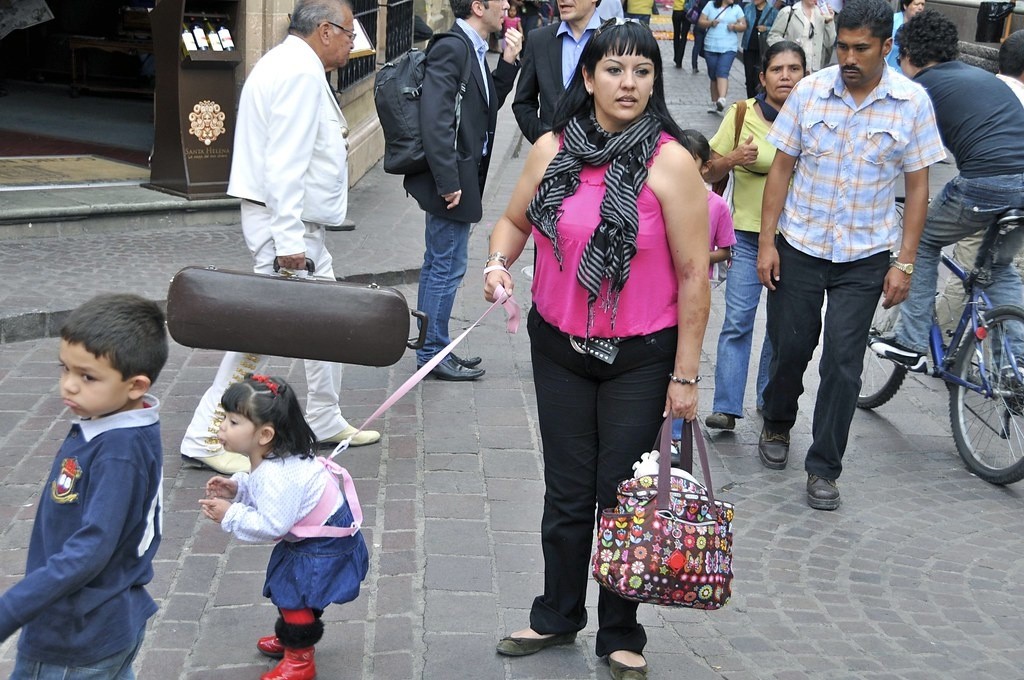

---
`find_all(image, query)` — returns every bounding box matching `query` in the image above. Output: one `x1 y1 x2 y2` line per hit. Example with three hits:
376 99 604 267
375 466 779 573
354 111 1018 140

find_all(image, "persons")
488 0 660 55
513 0 606 144
868 14 1024 385
702 41 807 430
672 0 926 112
0 293 169 680
199 372 368 680
671 128 738 466
937 31 1024 370
403 0 523 379
180 0 380 473
483 19 713 680
757 0 947 510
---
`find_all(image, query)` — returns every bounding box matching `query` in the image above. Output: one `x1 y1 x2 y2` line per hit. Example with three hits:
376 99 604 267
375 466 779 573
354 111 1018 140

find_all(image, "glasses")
586 18 650 54
895 50 905 66
317 22 358 41
808 22 815 39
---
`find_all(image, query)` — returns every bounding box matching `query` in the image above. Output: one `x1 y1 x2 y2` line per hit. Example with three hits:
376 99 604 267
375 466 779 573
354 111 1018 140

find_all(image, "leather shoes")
759 423 790 469
447 352 482 367
417 357 485 382
807 471 841 510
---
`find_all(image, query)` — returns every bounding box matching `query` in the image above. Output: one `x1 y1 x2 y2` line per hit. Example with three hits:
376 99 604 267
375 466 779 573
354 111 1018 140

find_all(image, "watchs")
893 261 915 274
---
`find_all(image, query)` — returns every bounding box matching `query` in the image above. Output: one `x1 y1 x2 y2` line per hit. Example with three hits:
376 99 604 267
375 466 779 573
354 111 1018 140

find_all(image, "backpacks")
374 33 473 175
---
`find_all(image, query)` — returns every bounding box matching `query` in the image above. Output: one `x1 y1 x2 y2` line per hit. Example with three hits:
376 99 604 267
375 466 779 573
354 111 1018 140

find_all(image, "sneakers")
866 335 926 372
1001 360 1024 383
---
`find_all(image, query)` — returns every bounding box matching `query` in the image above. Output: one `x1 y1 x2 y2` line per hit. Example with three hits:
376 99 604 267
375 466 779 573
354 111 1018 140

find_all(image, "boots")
258 608 323 657
260 619 324 680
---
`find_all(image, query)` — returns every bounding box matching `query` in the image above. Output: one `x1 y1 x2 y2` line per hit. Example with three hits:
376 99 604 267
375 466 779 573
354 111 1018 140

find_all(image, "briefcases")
166 257 429 367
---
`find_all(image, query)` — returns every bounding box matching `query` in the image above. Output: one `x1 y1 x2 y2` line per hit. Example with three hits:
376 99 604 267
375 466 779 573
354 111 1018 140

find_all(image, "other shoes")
708 101 719 112
180 453 251 474
606 651 647 680
497 630 578 656
716 98 726 112
313 424 381 450
692 68 699 73
706 410 734 430
675 63 682 68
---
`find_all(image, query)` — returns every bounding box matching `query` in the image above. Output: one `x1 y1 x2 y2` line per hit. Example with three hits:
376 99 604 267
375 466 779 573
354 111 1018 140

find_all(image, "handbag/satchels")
685 6 697 23
706 100 747 215
592 410 736 610
700 36 707 58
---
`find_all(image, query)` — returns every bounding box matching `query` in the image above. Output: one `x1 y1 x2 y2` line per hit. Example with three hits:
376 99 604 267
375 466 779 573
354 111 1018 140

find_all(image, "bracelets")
669 373 702 384
485 253 508 270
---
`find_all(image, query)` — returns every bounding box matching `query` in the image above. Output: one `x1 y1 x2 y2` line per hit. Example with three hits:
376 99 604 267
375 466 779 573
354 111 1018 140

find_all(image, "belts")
545 326 642 364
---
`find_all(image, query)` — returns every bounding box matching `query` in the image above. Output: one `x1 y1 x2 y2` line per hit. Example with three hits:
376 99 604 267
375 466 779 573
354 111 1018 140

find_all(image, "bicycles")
855 194 1024 487
